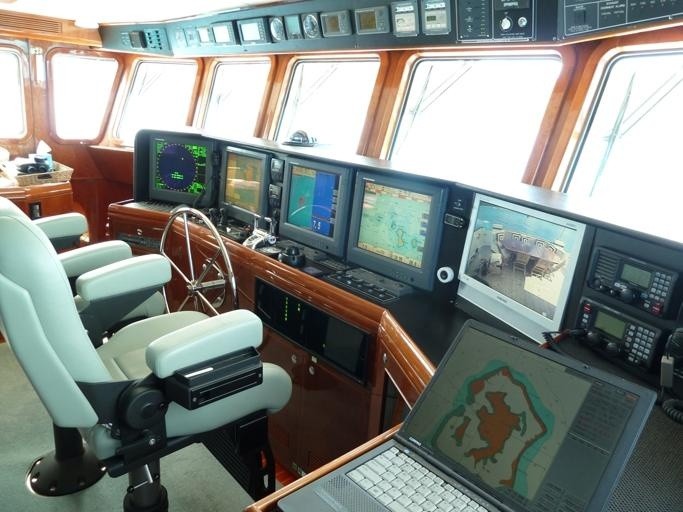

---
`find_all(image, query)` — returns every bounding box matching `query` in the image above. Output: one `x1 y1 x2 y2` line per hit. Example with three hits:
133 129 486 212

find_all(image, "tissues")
27 139 53 171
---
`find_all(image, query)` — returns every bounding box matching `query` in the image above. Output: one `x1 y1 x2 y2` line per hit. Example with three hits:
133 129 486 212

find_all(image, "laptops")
278 320 657 511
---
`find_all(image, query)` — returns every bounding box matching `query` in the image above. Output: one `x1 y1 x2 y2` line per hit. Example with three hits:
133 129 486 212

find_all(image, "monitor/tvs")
346 171 450 293
148 132 218 206
457 193 586 331
278 157 353 259
217 145 274 229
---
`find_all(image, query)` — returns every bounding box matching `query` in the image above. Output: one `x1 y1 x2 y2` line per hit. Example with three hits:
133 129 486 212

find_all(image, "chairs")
0 196 293 512
32 212 166 333
